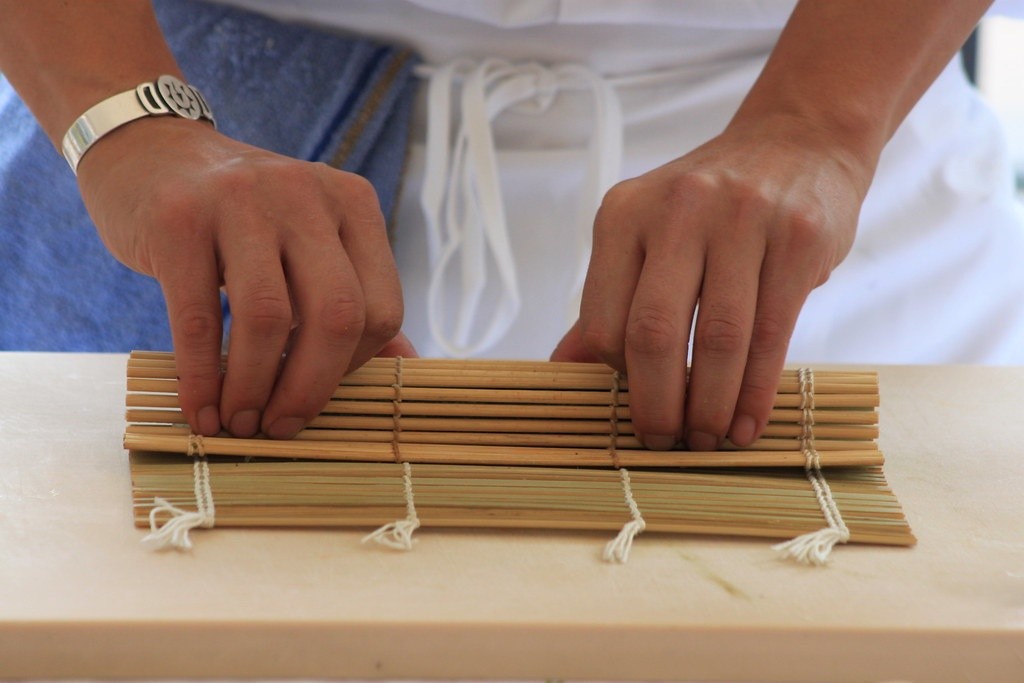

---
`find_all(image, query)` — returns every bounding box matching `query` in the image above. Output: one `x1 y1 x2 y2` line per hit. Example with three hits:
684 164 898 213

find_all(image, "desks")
0 349 1024 683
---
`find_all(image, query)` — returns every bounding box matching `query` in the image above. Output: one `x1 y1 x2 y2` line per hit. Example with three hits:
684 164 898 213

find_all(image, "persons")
0 0 1023 452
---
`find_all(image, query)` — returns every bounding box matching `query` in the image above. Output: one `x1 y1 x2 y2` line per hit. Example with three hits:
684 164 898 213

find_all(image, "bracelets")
60 75 217 175
